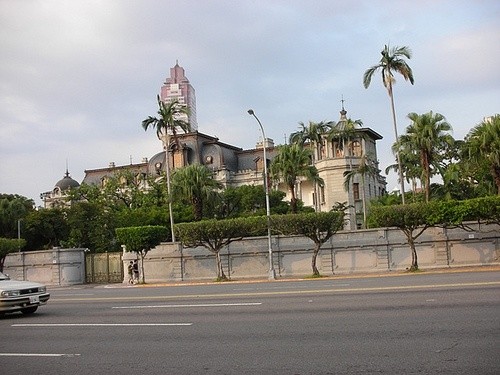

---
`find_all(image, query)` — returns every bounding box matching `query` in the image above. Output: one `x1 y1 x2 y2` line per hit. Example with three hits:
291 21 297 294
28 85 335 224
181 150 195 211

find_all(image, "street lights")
246 108 275 280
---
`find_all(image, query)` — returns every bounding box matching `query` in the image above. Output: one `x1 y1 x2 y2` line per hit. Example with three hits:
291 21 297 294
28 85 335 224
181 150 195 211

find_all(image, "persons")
132 259 140 284
127 261 133 285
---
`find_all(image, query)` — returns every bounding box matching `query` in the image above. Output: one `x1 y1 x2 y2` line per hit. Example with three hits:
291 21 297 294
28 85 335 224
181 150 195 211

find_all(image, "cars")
0 272 50 315
